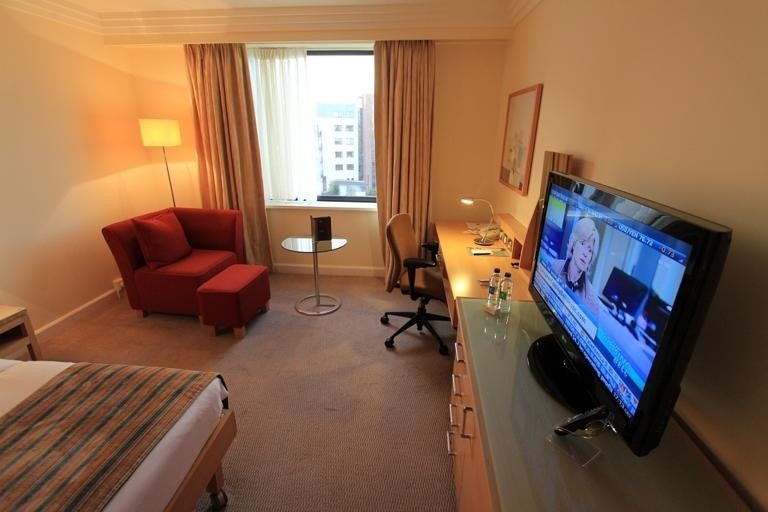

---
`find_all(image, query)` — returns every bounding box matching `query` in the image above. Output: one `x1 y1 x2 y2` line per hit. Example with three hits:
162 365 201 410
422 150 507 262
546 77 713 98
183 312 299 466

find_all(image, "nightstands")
0 305 45 363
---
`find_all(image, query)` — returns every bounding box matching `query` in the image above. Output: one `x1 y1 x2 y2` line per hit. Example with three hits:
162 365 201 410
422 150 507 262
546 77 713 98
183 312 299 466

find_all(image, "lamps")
137 116 183 207
460 198 495 246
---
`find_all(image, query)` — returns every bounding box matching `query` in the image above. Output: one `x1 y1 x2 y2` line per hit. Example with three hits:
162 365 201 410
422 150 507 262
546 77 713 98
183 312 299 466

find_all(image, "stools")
196 263 269 337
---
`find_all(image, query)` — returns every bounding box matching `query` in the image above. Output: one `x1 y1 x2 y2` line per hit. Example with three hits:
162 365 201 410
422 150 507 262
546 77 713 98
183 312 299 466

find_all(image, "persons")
470 248 506 254
553 211 603 332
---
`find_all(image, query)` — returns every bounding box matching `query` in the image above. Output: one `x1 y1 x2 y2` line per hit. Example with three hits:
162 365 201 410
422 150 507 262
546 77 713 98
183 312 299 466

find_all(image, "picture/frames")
498 82 542 197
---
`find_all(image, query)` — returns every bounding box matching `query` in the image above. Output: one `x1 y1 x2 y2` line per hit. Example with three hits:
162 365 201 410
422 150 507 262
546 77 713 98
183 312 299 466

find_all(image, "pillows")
135 211 193 267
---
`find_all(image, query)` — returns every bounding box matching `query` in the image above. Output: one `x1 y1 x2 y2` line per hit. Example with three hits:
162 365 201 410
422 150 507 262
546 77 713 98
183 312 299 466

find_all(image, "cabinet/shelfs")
447 298 752 512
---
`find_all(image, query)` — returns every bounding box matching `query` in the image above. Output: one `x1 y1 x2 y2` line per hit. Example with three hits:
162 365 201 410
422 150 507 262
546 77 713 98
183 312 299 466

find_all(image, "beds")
0 354 234 511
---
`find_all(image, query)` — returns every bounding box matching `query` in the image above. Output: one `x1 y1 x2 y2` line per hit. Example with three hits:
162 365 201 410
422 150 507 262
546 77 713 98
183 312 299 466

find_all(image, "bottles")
486 267 501 309
499 271 513 314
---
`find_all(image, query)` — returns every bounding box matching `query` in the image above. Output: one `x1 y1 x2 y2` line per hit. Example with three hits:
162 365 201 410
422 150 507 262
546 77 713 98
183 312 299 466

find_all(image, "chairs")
102 206 245 327
376 211 458 356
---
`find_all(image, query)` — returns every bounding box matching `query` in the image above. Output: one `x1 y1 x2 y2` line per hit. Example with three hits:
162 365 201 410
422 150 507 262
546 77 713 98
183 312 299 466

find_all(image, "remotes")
554 404 610 436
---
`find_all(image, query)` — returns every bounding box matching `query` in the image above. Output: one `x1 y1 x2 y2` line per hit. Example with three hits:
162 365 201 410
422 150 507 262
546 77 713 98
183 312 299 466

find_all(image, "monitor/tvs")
528 171 732 456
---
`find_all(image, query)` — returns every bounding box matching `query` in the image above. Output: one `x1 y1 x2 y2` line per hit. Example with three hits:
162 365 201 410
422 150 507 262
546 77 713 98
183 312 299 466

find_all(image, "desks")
435 206 538 327
282 236 348 317
